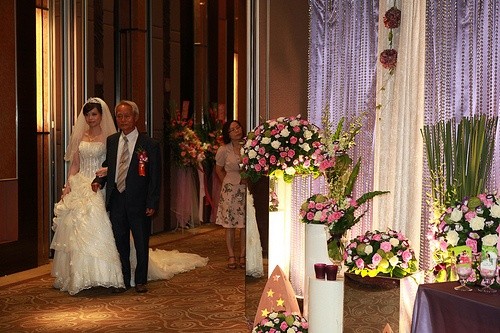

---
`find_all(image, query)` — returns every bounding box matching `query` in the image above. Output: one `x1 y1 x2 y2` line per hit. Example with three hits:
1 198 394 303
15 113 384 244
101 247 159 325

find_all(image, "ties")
117 137 130 193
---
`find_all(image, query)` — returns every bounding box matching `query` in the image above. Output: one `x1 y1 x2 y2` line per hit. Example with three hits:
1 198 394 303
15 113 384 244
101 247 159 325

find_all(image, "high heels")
239 257 246 268
228 256 236 268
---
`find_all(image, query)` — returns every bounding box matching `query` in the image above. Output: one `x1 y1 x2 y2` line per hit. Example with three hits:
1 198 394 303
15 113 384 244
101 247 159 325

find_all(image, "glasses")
229 126 240 133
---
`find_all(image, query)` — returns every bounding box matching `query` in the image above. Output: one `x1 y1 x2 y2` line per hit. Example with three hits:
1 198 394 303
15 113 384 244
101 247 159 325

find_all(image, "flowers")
135 147 148 162
204 119 232 166
252 310 309 333
164 109 204 167
422 166 500 290
235 107 417 279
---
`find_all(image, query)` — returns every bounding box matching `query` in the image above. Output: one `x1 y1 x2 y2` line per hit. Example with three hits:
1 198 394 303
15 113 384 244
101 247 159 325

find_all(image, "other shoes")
113 287 128 293
135 283 147 293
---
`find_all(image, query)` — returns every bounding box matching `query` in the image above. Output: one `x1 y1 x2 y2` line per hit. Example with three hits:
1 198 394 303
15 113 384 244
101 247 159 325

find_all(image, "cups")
326 265 338 281
314 263 326 280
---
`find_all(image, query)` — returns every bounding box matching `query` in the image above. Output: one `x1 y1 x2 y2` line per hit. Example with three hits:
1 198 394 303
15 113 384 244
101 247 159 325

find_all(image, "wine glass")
454 262 473 292
479 257 497 293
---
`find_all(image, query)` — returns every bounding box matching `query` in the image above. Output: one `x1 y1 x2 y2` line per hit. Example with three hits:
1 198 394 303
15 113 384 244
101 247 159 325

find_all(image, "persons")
215 118 252 270
53 96 122 292
90 100 162 294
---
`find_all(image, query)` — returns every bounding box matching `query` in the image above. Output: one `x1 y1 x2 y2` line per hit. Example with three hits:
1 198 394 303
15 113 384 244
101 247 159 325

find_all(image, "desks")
410 281 500 333
